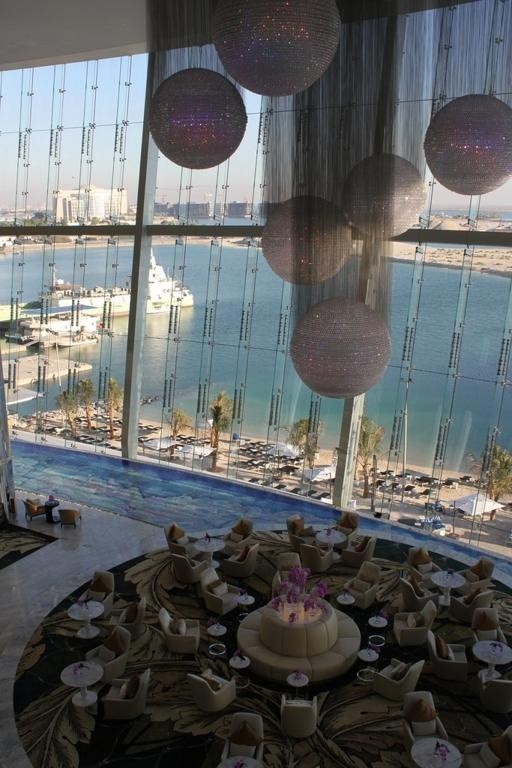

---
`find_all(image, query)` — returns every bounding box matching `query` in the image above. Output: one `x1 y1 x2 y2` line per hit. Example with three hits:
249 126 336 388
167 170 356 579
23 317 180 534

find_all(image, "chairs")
78 509 512 768
46 413 481 519
21 498 46 520
59 509 82 528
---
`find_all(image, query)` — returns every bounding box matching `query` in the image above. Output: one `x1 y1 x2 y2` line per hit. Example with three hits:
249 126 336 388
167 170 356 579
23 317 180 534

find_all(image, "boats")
39 278 132 318
5 313 102 346
148 249 195 308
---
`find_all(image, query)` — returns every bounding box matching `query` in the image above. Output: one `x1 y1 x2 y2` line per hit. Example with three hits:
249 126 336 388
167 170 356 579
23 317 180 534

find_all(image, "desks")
67 599 105 640
60 660 104 706
45 501 60 524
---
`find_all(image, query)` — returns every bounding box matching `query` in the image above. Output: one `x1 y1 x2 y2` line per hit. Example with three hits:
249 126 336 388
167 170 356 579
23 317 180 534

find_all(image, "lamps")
343 151 424 237
289 300 393 401
208 0 341 94
260 190 352 284
149 67 249 168
422 91 512 196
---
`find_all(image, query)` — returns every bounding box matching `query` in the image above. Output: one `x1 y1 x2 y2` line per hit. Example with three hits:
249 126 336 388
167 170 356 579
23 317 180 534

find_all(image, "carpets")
0 523 59 569
13 530 512 768
